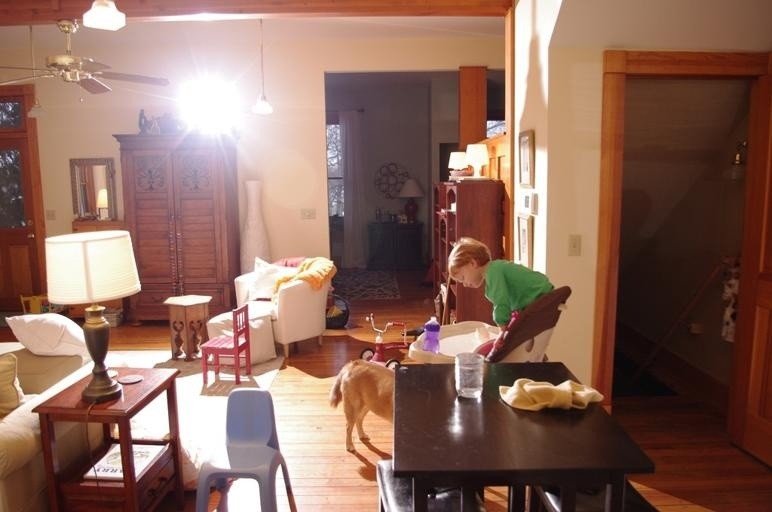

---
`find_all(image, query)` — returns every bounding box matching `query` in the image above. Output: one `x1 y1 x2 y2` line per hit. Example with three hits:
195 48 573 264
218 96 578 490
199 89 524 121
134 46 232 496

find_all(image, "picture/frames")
516 214 533 270
519 129 536 189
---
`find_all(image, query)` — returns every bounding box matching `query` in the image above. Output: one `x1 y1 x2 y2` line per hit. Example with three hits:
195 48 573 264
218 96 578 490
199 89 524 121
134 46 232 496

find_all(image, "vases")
242 180 270 272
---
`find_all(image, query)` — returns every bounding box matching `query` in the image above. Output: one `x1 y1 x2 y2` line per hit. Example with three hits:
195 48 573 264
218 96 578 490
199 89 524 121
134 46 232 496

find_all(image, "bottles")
423 316 439 354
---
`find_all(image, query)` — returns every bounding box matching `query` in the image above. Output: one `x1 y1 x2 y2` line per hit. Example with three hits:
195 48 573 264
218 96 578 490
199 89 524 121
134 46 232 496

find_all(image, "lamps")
25 26 50 120
398 179 425 224
44 231 143 402
466 143 489 179
80 0 127 31
723 137 748 182
252 18 273 116
447 151 469 176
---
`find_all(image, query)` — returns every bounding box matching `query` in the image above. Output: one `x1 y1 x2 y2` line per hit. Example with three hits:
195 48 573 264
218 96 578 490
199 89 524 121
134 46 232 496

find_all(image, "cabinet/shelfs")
370 223 427 270
433 182 506 327
111 132 241 327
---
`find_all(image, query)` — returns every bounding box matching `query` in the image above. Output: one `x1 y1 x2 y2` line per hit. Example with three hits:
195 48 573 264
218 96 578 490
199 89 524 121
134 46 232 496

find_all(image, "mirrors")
69 157 118 220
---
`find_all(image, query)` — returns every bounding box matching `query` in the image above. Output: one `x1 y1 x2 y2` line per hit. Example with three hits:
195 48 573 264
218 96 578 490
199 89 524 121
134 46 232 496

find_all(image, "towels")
498 378 603 412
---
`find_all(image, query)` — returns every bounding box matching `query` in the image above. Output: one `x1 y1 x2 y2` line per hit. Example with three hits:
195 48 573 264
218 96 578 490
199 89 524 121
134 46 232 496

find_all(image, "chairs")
195 386 298 511
200 304 252 384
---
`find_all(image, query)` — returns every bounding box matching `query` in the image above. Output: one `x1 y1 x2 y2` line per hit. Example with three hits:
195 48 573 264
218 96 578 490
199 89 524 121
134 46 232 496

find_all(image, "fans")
1 18 167 96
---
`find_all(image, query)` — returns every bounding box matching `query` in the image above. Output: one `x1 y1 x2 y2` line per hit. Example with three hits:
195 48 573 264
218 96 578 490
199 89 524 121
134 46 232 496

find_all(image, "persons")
448 236 554 333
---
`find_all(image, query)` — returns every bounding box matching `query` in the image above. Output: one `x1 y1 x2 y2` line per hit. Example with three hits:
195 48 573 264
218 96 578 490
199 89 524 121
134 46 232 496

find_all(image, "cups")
447 395 485 437
454 352 485 400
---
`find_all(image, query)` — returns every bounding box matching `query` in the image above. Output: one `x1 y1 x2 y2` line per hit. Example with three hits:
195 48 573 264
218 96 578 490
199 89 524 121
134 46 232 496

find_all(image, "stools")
379 456 482 511
531 478 657 512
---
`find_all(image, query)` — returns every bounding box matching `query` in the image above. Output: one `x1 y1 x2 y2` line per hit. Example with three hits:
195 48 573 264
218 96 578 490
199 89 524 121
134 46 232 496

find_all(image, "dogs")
330 360 396 452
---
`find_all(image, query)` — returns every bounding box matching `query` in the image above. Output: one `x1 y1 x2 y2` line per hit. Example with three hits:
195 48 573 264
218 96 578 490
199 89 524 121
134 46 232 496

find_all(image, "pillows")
6 311 88 358
251 256 296 297
1 355 25 410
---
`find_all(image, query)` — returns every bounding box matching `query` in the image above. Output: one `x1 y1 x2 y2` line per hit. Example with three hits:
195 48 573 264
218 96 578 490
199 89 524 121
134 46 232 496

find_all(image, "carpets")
332 268 399 299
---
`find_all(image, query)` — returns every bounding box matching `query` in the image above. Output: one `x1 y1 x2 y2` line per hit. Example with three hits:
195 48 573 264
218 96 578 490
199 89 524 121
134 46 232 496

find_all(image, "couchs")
233 258 336 360
206 311 276 366
0 344 114 510
